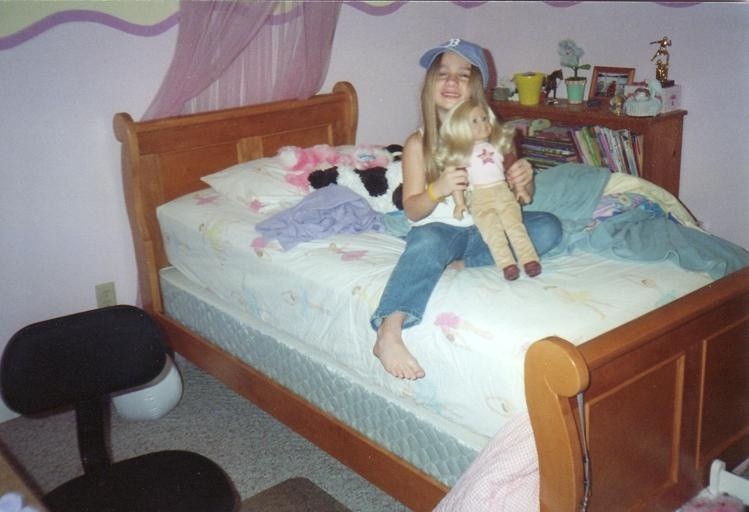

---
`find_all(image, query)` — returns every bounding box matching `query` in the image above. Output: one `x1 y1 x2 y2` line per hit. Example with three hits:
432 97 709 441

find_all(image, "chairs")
0 304 236 512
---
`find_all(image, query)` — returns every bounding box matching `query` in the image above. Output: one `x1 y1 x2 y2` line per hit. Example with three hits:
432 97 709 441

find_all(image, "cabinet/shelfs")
486 96 687 198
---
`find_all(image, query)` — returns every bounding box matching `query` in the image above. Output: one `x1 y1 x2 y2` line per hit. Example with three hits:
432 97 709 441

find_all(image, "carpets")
0 351 414 512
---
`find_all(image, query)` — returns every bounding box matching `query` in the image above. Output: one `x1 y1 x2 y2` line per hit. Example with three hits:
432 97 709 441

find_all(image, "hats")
420 39 489 89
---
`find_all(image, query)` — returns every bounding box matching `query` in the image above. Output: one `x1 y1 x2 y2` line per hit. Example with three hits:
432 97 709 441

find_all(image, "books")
513 117 643 177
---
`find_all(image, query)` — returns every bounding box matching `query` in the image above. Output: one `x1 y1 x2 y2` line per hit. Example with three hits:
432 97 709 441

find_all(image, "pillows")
199 142 386 216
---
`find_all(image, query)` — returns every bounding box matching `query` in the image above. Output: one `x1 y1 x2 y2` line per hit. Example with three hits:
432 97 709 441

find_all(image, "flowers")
556 39 591 81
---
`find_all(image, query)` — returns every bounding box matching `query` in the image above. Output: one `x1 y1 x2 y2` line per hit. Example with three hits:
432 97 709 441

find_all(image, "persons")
435 98 541 280
369 38 563 380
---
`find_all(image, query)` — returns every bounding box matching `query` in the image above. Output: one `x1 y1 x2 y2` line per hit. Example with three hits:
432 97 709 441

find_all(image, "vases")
564 77 587 105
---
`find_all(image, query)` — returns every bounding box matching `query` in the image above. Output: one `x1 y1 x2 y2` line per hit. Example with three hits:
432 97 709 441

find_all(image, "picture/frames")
588 65 635 104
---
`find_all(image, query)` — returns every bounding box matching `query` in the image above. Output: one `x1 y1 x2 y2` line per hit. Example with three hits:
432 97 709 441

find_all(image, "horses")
542 69 563 100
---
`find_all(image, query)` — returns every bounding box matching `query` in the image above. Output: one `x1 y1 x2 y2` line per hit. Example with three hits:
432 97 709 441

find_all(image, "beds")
113 81 749 512
